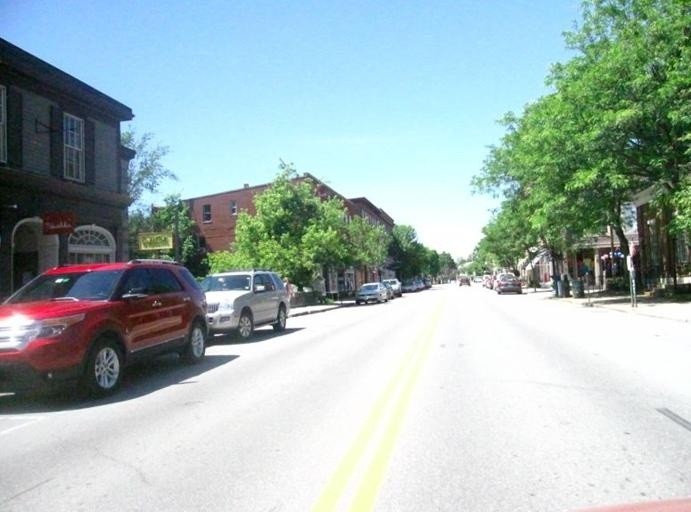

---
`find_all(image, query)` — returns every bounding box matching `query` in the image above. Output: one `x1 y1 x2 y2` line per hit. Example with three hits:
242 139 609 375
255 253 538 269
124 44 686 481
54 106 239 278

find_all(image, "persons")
317 275 327 304
283 276 295 298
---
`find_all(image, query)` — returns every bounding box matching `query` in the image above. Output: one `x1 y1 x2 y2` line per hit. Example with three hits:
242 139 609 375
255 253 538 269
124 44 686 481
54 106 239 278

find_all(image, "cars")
483 273 522 294
354 278 402 304
402 277 432 292
459 277 470 286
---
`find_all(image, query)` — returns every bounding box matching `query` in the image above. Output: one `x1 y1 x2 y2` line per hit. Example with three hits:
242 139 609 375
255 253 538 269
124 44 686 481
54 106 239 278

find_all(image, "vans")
200 269 287 342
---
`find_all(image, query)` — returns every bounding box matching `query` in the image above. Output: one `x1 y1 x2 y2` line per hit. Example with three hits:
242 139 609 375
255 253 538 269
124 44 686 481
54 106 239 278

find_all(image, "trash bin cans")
572 279 585 298
556 280 569 297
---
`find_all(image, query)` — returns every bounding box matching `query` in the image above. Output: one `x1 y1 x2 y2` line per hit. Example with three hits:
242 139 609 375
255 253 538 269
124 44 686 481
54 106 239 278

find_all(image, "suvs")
0 259 208 399
